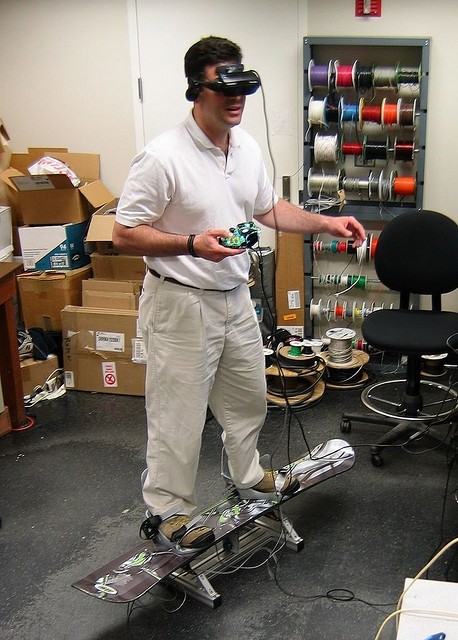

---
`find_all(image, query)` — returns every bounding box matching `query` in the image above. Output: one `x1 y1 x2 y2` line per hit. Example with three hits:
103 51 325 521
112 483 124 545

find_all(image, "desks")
1 261 28 431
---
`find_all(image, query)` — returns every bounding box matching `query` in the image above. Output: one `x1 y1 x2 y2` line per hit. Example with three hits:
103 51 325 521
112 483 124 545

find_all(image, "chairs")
340 211 457 464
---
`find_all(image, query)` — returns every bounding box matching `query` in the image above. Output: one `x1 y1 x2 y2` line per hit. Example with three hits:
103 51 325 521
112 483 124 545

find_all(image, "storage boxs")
81 278 142 308
18 224 91 270
61 305 146 399
90 253 146 281
2 152 114 223
84 199 125 242
16 264 92 328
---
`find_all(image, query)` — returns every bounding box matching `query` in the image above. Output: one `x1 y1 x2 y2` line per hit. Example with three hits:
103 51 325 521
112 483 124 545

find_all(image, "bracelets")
186 233 201 259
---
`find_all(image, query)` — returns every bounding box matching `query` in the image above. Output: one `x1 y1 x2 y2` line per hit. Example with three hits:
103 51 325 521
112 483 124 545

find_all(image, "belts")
148 267 240 293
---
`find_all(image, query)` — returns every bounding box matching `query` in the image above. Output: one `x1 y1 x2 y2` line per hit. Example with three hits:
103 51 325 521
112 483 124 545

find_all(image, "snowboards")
70 438 356 603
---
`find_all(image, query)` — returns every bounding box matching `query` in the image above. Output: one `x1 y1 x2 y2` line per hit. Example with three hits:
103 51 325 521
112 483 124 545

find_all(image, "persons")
111 35 364 548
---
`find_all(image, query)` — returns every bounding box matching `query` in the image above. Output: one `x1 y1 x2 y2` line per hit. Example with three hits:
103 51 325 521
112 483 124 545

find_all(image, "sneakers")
229 471 300 494
158 514 216 548
